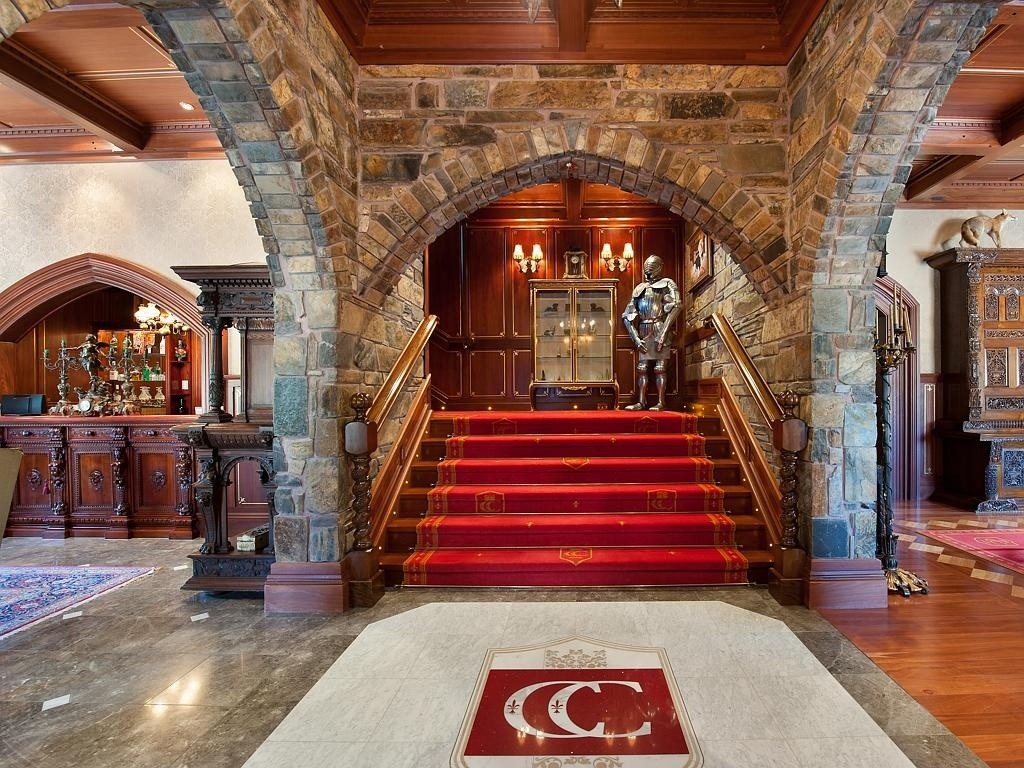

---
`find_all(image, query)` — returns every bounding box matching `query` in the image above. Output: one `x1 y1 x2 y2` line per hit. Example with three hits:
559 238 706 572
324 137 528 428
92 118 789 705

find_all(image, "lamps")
135 300 191 337
513 242 544 275
600 242 635 272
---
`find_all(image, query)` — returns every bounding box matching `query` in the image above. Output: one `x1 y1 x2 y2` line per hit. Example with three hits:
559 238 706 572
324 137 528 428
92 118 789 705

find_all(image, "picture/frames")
685 226 713 294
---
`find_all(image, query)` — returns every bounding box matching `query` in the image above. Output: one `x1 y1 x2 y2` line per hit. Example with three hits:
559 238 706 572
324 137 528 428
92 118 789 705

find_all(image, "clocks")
563 250 589 280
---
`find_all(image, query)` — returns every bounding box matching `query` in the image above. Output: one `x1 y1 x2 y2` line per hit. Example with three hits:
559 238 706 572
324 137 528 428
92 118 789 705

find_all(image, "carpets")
0 566 163 641
915 528 1024 575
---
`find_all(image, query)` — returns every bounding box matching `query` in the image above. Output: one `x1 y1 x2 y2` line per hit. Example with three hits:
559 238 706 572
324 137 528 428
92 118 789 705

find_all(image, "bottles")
178 399 185 415
110 361 118 380
142 360 150 381
131 366 140 382
154 362 162 374
113 384 121 402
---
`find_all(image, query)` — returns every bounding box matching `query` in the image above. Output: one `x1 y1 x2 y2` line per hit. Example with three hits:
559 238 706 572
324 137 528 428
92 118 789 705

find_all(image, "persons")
621 254 682 411
110 334 118 352
58 334 109 380
123 336 132 351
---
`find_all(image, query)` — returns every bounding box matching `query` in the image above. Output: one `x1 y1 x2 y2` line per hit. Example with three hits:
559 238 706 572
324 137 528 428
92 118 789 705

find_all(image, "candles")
876 310 879 339
888 283 912 343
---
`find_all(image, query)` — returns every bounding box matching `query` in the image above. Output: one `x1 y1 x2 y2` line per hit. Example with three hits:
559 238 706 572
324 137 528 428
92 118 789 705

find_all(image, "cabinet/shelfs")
92 321 193 415
527 277 620 408
